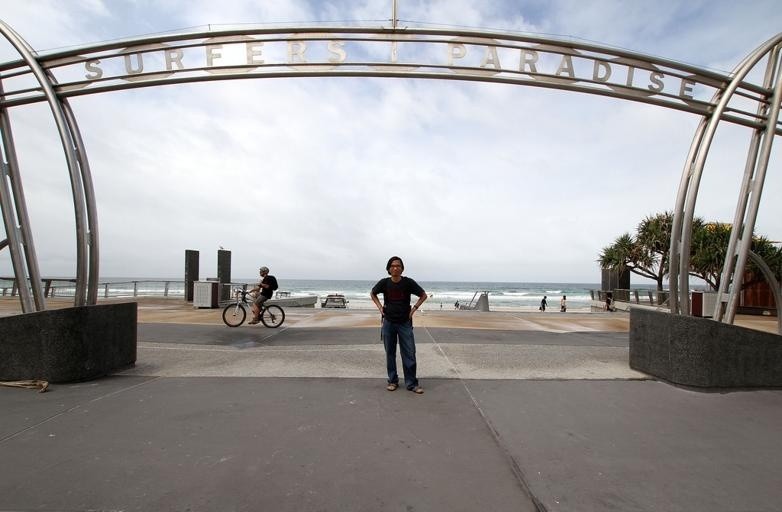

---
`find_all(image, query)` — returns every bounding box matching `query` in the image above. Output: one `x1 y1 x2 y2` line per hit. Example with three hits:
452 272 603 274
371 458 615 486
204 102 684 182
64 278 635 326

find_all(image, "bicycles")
222 285 285 327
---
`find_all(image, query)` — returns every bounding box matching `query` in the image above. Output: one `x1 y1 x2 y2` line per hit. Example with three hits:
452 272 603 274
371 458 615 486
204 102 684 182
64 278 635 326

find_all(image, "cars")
320 294 349 308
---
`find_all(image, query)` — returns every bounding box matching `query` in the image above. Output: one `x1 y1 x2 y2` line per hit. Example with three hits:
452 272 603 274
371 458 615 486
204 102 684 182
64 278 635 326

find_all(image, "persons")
454 300 459 310
541 296 547 312
561 296 567 312
370 256 427 394
604 293 614 311
248 267 278 324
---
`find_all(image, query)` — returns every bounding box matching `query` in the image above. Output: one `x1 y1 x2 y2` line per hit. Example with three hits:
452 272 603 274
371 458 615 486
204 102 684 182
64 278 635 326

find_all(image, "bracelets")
414 305 418 309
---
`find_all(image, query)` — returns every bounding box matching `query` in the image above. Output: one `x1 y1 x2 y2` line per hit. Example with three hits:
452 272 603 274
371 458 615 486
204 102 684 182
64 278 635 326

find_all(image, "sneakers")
248 320 259 324
387 383 398 391
415 388 424 394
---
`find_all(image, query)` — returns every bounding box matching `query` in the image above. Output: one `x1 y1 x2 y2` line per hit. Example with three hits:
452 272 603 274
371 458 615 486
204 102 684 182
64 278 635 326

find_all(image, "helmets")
260 267 269 274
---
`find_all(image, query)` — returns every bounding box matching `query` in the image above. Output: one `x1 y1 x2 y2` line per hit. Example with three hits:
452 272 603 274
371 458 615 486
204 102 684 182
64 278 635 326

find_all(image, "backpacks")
268 275 278 290
